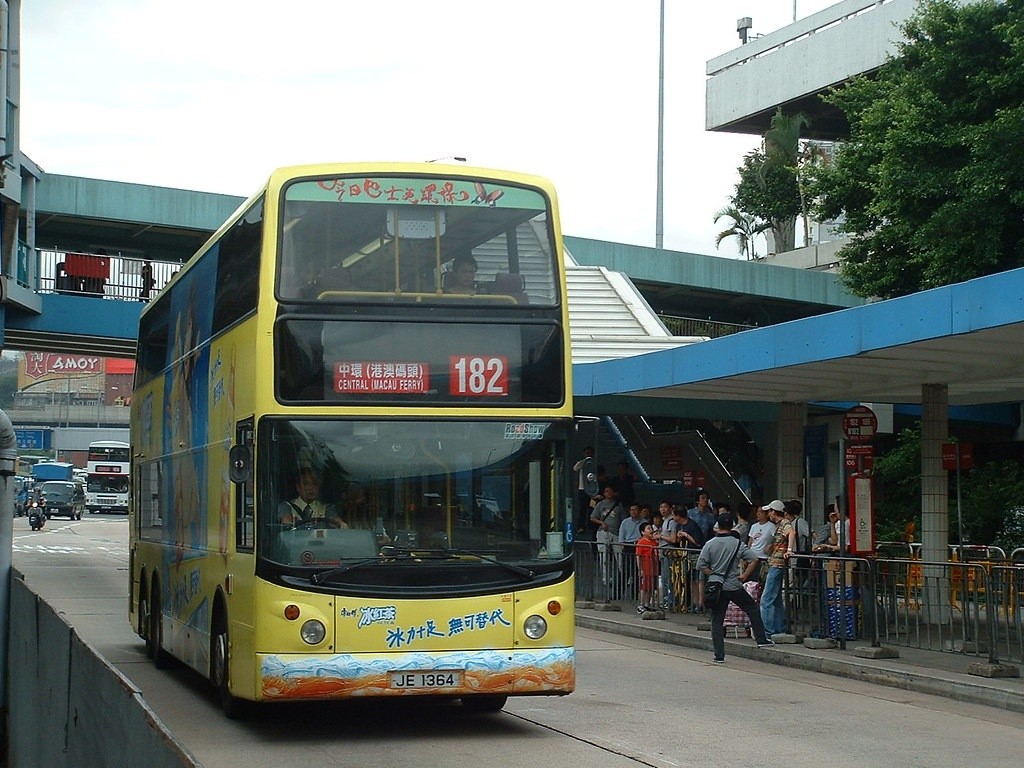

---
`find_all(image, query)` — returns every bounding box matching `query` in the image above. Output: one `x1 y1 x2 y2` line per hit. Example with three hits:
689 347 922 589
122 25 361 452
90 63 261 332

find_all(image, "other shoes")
638 602 702 615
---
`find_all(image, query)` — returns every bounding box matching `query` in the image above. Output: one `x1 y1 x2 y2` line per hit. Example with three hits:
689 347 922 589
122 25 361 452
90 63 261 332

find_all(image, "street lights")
81 386 118 428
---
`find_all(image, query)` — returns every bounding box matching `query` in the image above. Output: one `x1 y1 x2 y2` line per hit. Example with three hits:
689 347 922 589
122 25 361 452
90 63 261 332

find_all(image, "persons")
278 466 349 530
443 254 478 296
15 487 46 521
140 260 156 303
574 447 860 663
174 288 197 543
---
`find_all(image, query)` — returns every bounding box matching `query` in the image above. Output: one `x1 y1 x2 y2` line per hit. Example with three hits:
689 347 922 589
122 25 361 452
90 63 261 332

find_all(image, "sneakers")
713 655 725 662
758 640 775 646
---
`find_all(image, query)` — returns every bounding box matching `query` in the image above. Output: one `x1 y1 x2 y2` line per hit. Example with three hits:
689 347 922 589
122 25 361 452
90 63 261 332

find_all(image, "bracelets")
787 547 792 552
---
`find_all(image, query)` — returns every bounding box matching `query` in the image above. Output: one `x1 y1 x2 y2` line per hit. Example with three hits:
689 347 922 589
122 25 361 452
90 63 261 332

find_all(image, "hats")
761 500 785 511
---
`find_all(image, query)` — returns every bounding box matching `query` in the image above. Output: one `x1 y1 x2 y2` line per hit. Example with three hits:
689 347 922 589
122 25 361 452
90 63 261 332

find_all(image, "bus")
127 162 599 718
85 441 130 513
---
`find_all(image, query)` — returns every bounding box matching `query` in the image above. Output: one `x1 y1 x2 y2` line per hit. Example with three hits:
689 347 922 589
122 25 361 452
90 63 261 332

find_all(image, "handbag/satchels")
704 582 722 609
795 551 811 576
764 543 773 556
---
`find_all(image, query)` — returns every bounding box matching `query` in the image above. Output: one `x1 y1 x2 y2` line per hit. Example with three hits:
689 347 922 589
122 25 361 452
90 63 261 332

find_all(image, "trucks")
31 461 74 506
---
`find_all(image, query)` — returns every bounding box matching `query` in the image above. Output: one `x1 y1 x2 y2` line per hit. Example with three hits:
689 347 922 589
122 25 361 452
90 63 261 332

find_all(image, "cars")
14 476 35 518
73 468 87 494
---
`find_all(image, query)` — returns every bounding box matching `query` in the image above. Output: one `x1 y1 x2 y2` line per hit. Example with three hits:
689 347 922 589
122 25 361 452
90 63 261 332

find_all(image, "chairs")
310 259 530 304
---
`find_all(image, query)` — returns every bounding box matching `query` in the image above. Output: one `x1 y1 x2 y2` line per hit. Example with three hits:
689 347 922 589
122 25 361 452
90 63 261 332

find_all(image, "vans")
40 481 86 520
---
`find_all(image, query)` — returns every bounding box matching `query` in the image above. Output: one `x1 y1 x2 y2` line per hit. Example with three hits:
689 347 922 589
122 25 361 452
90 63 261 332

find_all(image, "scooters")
28 496 47 532
